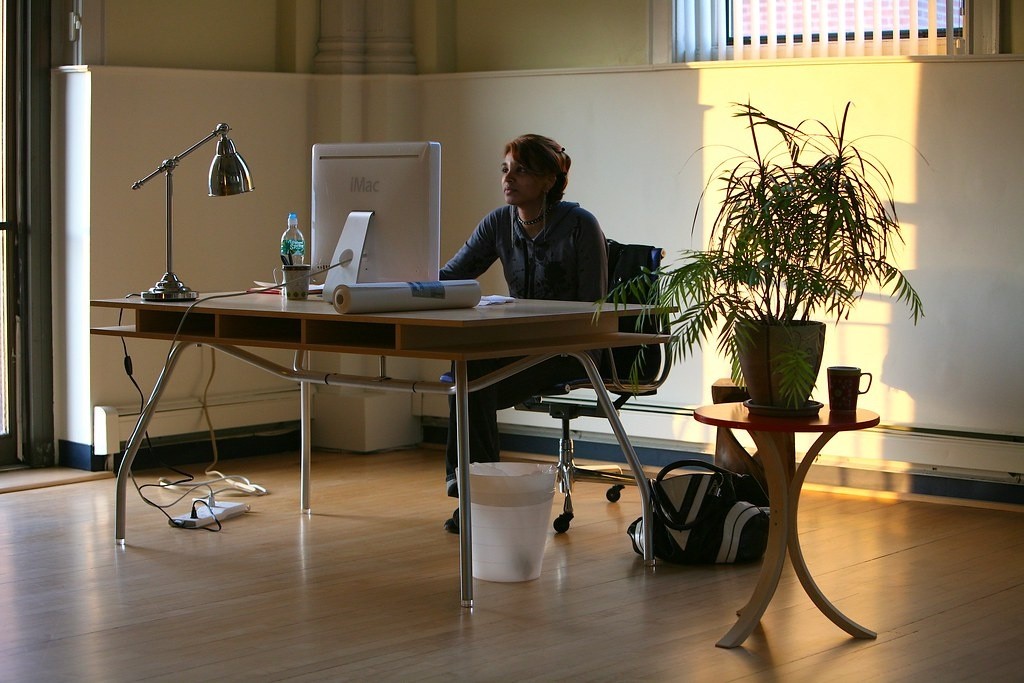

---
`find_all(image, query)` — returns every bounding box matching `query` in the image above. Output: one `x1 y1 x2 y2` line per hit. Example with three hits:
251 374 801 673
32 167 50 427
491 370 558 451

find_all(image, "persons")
438 134 611 531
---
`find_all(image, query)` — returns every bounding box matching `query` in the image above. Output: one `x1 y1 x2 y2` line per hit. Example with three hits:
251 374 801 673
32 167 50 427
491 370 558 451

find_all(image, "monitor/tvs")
311 141 442 304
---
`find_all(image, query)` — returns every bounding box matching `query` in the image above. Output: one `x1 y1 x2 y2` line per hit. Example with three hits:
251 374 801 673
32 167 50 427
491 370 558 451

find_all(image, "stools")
711 378 796 499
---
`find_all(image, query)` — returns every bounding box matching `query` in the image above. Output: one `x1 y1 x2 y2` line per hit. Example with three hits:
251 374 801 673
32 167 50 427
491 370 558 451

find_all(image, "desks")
89 288 681 609
693 404 880 648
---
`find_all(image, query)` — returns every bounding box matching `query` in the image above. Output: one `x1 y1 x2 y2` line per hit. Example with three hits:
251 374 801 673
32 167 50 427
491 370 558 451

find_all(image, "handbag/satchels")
627 460 769 565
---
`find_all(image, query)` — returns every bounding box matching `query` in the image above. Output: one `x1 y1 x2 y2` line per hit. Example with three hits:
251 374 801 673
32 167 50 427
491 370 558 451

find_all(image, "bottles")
281 212 306 296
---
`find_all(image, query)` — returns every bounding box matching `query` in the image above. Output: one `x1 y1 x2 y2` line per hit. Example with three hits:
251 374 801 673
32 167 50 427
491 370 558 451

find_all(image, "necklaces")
518 202 552 225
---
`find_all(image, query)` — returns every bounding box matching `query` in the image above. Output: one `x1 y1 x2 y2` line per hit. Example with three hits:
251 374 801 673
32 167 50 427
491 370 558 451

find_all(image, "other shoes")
444 507 459 534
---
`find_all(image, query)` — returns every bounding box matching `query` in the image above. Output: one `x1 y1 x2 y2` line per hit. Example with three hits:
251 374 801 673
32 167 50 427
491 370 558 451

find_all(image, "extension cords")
169 500 247 529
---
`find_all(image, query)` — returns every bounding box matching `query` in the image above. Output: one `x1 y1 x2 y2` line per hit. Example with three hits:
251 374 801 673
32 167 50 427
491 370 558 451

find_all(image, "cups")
281 264 312 300
826 366 872 412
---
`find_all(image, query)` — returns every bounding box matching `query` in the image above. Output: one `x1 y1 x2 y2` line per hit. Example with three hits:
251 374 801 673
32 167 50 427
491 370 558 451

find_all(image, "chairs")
513 240 672 533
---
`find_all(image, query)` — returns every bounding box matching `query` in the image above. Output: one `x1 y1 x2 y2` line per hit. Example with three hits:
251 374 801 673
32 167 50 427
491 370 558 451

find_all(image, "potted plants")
591 99 935 404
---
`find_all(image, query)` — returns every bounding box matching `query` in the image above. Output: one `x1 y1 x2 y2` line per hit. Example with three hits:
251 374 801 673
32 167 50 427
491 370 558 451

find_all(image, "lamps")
131 122 257 302
